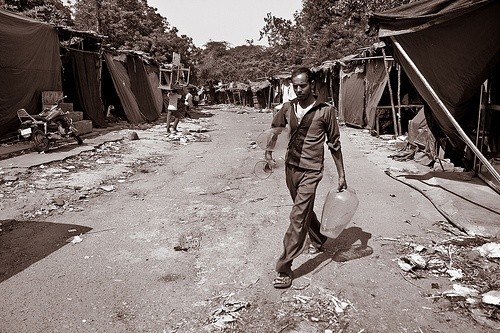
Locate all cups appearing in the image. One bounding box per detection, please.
[257,127,291,152]
[255,157,286,180]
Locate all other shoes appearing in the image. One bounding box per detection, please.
[309,242,321,253]
[272,271,292,288]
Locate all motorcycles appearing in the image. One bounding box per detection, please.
[16,96,83,152]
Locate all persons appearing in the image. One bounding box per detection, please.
[194,92,209,111]
[183,88,193,119]
[166,89,182,134]
[263,66,348,288]
